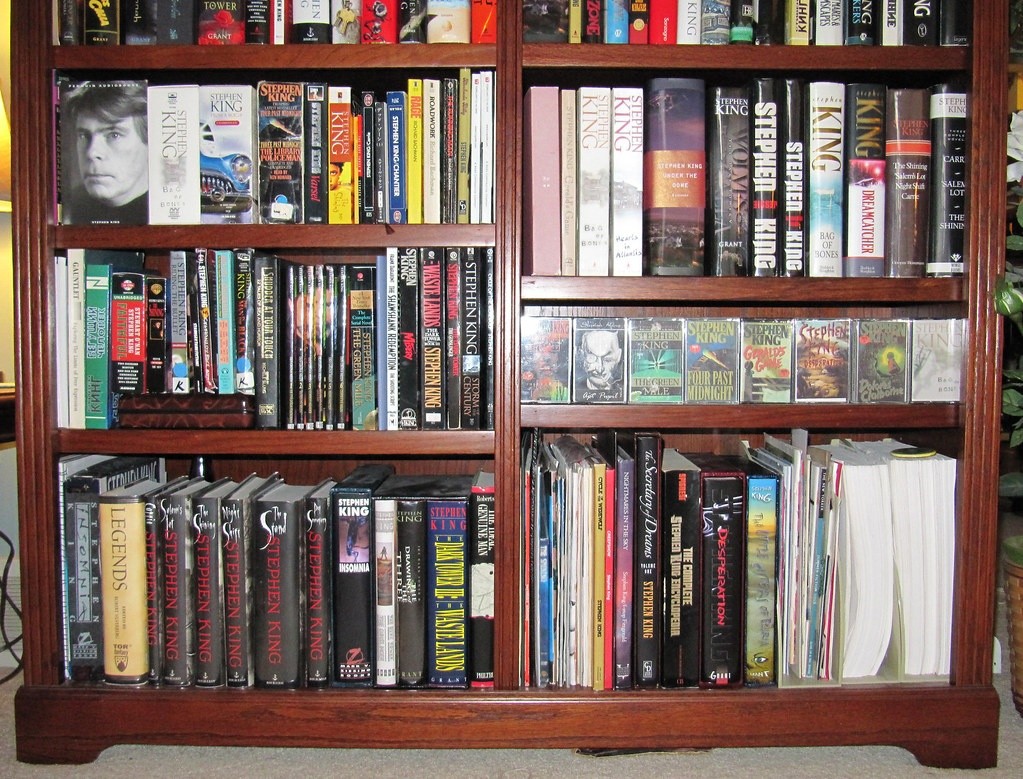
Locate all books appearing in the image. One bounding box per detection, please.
[54,0,968,691]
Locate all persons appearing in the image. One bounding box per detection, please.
[329,162,345,191]
[64,80,149,224]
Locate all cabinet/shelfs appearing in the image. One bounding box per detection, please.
[10,0,1005,767]
